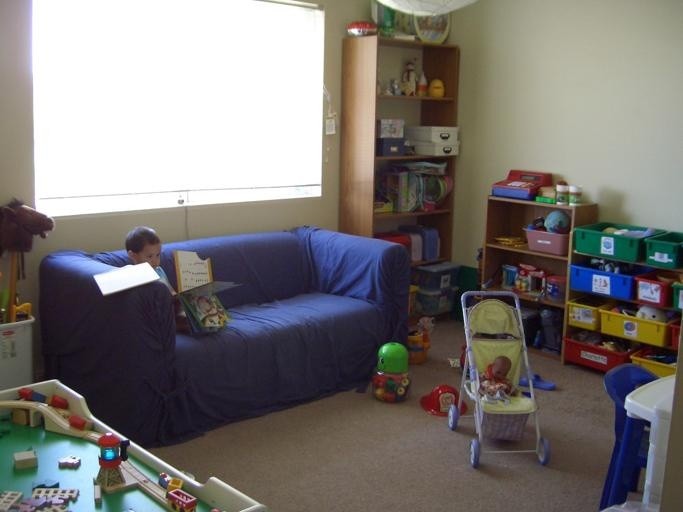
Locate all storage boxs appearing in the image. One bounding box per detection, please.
[370,0,450,44]
[561,222,683,377]
[523,225,569,256]
[409,261,461,317]
[0,314,35,415]
[377,118,461,157]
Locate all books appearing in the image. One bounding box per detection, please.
[172,249,241,337]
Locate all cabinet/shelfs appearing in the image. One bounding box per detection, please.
[480,195,599,366]
[338,35,461,326]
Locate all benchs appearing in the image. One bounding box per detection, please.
[40,225,410,450]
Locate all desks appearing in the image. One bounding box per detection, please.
[607,374,675,508]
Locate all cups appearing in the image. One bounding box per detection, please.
[555,184,569,205]
[568,185,582,207]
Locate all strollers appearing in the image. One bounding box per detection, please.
[443,288,556,469]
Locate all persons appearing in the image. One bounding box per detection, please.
[125,226,187,335]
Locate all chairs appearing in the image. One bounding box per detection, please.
[598,363,659,512]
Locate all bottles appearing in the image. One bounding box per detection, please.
[416,71,428,97]
